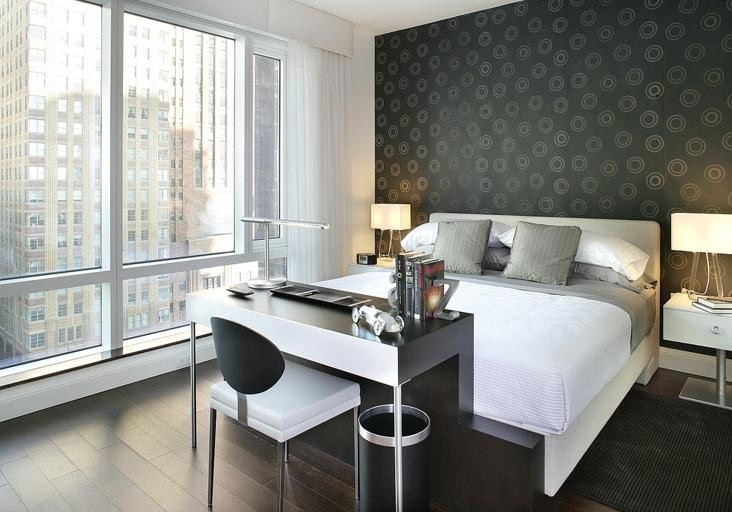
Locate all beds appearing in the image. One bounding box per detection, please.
[306,208,658,495]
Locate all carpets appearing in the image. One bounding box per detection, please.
[560,388,731,511]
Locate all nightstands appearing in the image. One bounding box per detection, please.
[346,257,396,275]
[662,293,732,409]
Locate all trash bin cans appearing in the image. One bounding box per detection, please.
[358,404,431,511]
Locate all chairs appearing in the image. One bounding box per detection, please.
[203,314,362,512]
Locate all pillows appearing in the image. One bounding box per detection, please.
[504,221,584,287]
[430,218,492,277]
[497,227,652,279]
[403,219,512,251]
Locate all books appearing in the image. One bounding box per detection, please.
[398,251,444,320]
[692,301,732,314]
[697,297,732,309]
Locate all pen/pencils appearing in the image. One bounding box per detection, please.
[707,298,732,303]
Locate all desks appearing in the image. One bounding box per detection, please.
[187,276,474,512]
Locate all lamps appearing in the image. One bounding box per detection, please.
[240,215,329,290]
[670,212,732,306]
[368,201,411,262]
